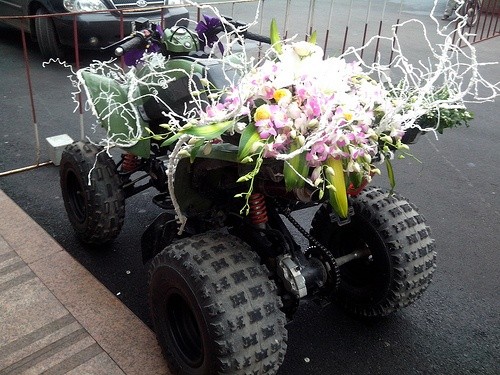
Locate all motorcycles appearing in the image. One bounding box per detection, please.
[58,13,438,375]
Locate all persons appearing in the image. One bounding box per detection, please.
[442,0,483,20]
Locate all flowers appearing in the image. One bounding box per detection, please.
[126,18,475,219]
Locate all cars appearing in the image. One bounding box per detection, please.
[0,0,187,64]
